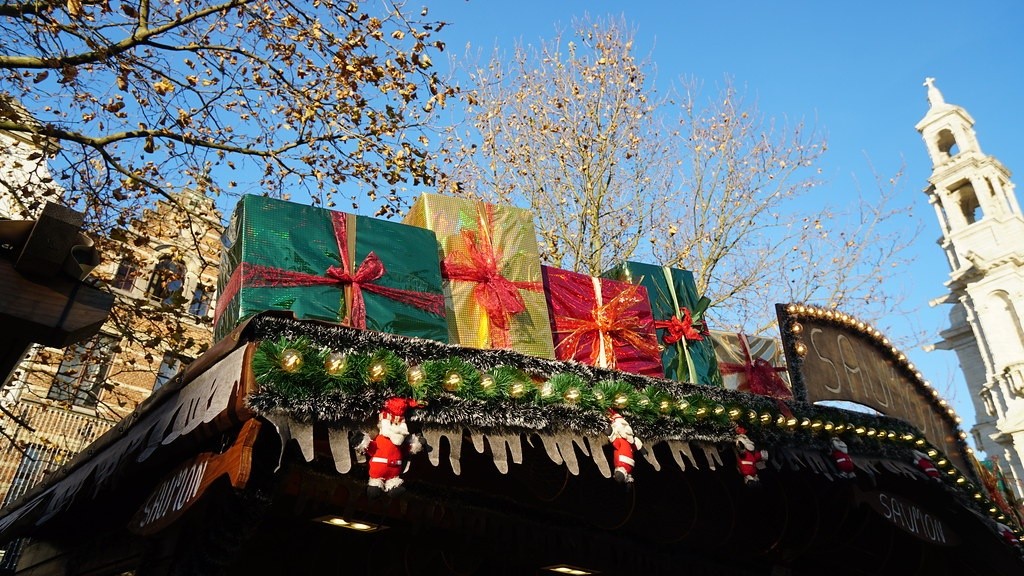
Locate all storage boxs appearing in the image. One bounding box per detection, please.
[212,191,790,399]
